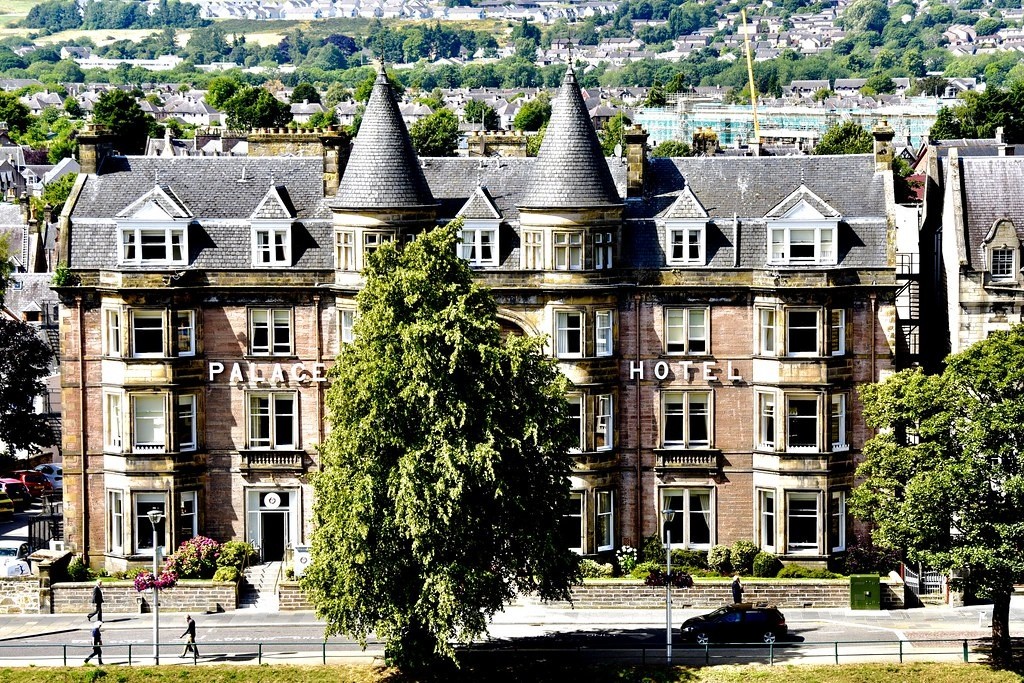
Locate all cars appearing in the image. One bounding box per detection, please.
[679,602,787,648]
[0,463,62,517]
[0,539,35,575]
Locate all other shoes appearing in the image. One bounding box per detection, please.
[193,656,199,659]
[178,656,184,658]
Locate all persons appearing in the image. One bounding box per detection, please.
[84,624,104,666]
[87,580,103,623]
[179,614,200,659]
[732,575,744,605]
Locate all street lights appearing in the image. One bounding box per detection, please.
[146,507,163,665]
[661,509,676,666]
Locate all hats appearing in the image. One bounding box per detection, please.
[733,575,739,581]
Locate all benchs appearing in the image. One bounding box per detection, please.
[48,522,63,540]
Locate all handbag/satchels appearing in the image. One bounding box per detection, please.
[91,601,97,613]
[188,641,195,651]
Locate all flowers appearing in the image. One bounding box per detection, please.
[135,569,179,591]
[646,567,693,590]
